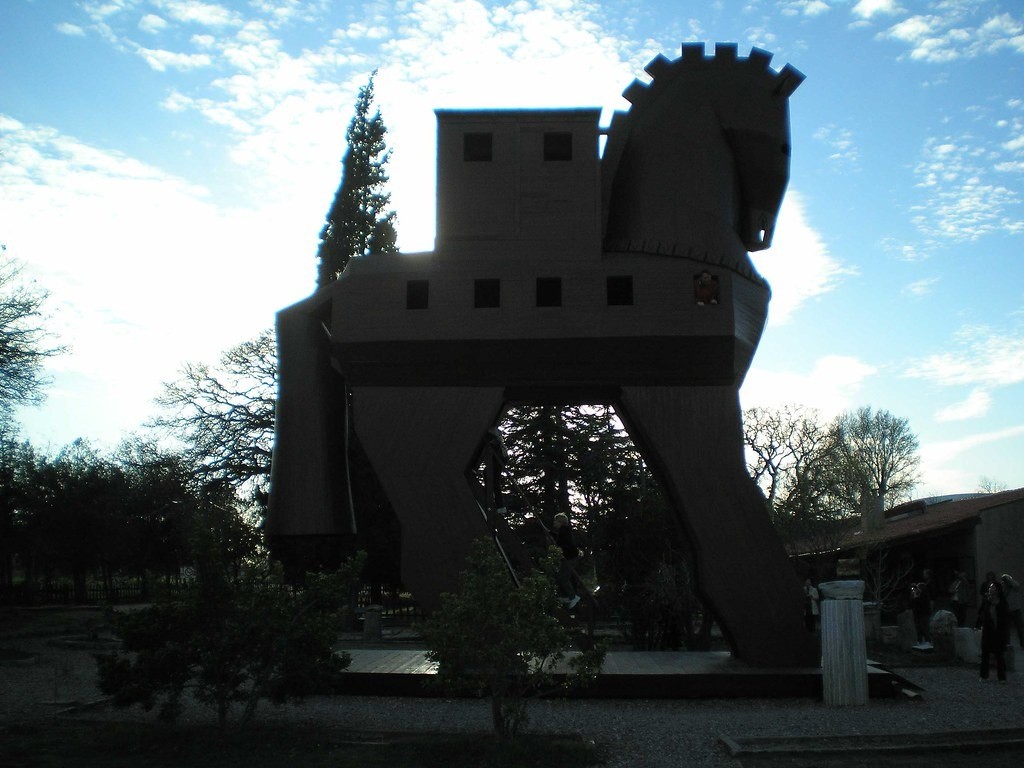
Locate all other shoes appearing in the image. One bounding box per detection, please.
[496,497,502,507]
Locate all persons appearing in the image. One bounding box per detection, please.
[802,577,819,632]
[909,569,1024,684]
[543,513,583,604]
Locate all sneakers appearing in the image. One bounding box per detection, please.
[569,595,581,610]
